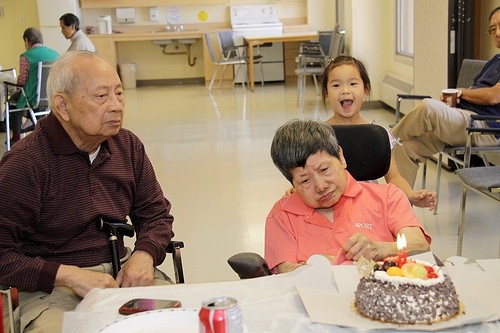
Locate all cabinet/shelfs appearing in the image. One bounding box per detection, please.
[90,38,117,73]
[202,38,234,89]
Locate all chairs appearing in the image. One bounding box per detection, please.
[228,124,392,279]
[294,24,344,108]
[389,59,500,256]
[0,61,54,152]
[0,241,185,333]
[205,30,263,91]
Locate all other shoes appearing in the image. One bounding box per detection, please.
[20,123,36,133]
[3,139,18,147]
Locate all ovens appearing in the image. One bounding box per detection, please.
[232,28,284,83]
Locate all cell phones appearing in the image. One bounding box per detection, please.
[118,298,182,315]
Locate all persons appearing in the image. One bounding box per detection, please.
[0,50,175,333]
[60,13,96,53]
[390,7,500,190]
[264,120,432,274]
[10,27,59,144]
[322,56,438,211]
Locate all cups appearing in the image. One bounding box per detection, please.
[442,88,459,108]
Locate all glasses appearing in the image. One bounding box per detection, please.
[488,22,500,35]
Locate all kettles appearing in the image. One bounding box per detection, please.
[96,15,113,34]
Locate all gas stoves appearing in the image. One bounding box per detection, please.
[230,4,282,28]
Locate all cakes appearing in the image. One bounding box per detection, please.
[355,257,459,324]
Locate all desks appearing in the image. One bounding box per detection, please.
[66,266,500,333]
[243,31,318,92]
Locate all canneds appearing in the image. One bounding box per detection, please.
[198,295,244,333]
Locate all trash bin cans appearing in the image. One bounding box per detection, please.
[119,63,136,90]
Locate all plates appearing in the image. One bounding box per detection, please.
[96,306,248,333]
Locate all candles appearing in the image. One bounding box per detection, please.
[401,233,407,264]
[397,233,402,268]
